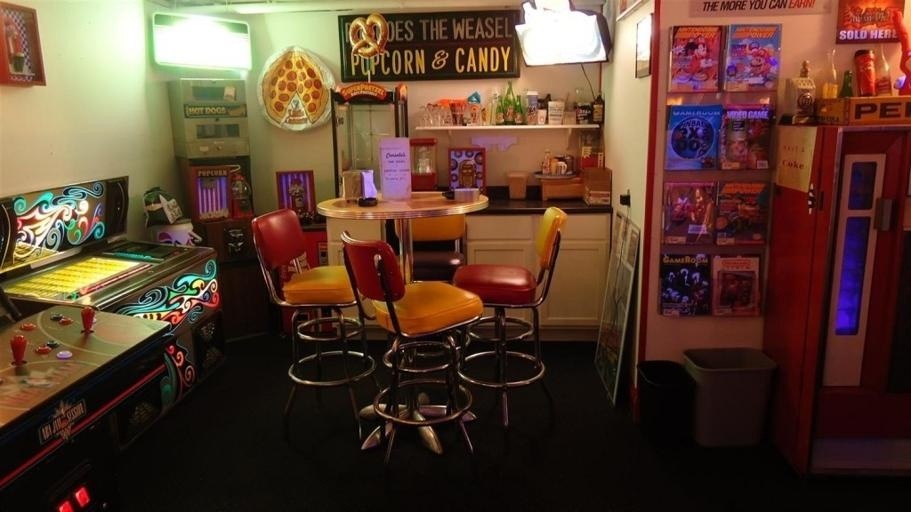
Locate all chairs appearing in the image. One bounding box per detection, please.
[386,208,474,362]
[447,202,570,455]
[249,207,388,446]
[335,228,486,463]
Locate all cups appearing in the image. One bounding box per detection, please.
[507,171,528,200]
[416,100,498,128]
[854,49,877,98]
[536,109,548,124]
[524,88,539,126]
[548,101,564,126]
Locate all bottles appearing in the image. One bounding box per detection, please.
[872,42,894,98]
[819,47,840,99]
[538,148,575,177]
[494,80,529,126]
[573,87,592,124]
[591,92,605,124]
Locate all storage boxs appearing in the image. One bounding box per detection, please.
[580,165,613,208]
[813,94,910,126]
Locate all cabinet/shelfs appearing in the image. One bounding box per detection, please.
[324,214,391,330]
[771,119,910,481]
[468,209,615,345]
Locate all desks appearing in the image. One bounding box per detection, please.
[315,188,490,465]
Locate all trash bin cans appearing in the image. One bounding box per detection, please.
[637,360,690,429]
[682,348,777,448]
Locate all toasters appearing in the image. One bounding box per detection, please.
[835,68,854,99]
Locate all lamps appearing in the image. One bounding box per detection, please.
[150,9,256,76]
[511,0,614,70]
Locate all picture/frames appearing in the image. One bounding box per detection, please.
[0,2,47,88]
[634,12,654,80]
[447,145,489,197]
